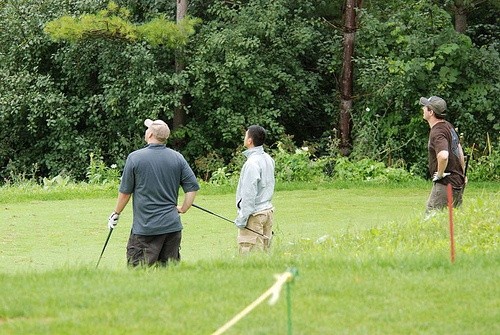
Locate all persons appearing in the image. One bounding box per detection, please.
[234,125,275,254]
[420,96,469,220]
[108,119,200,269]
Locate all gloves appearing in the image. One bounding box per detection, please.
[233,216,248,229]
[108,211,119,230]
[464,176,468,185]
[432,171,451,182]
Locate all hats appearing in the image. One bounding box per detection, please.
[144,119,170,141]
[420,96,448,115]
